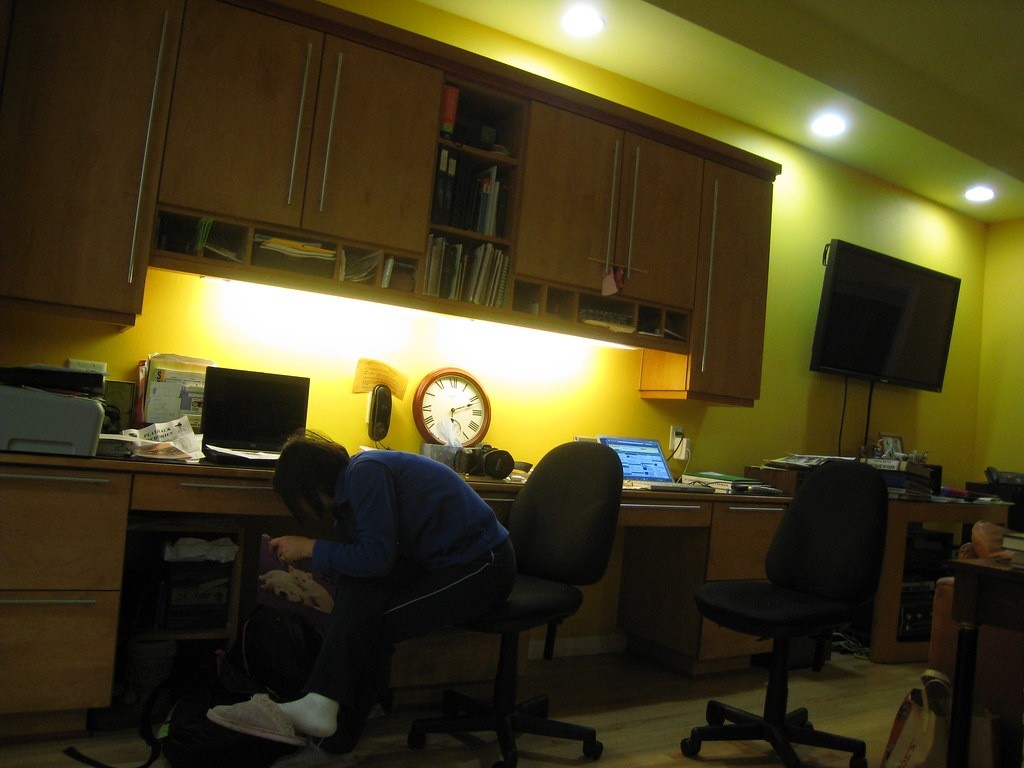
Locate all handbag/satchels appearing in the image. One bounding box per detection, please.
[880,669,997,768]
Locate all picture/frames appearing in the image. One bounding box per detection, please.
[878,432,905,458]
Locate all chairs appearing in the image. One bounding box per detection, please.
[404,441,623,768]
[680,459,891,768]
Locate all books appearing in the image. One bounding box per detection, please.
[1000,529,1024,567]
[253,232,336,263]
[418,231,509,307]
[433,147,500,236]
[436,82,461,141]
[681,470,764,495]
[137,353,208,428]
[254,533,337,635]
[760,452,1003,504]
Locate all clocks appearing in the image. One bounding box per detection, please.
[412,369,492,445]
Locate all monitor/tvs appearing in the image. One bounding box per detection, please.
[809,238,962,394]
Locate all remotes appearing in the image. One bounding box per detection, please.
[747,485,782,496]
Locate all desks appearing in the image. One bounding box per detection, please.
[0,446,788,733]
[945,556,1024,768]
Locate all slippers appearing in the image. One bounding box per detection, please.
[207,693,310,746]
[270,740,361,768]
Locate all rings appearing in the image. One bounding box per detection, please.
[280,555,286,561]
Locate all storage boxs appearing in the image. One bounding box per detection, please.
[143,552,234,630]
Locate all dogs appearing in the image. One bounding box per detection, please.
[259,569,301,603]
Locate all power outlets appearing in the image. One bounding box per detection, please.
[670,426,685,448]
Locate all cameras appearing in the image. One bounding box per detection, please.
[454,444,514,480]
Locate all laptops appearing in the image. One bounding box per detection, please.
[202,366,310,466]
[596,436,716,494]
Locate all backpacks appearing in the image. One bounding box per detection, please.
[142,608,325,768]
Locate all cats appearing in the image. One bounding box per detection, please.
[287,565,334,614]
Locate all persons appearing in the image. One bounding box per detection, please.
[207,430,518,768]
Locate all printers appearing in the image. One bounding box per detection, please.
[0,363,133,460]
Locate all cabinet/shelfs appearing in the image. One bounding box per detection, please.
[744,465,944,497]
[0,0,169,328]
[152,0,443,301]
[961,481,1024,544]
[414,34,534,322]
[500,63,700,345]
[686,135,780,400]
[867,497,1008,659]
